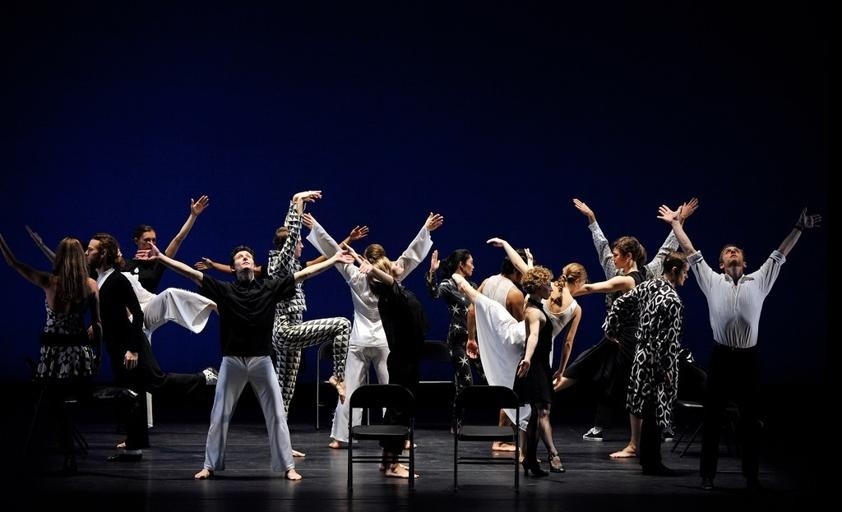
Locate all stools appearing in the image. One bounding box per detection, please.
[666,395,744,458]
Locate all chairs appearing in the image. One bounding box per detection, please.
[419,341,458,384]
[444,382,523,491]
[342,381,418,497]
[314,337,374,430]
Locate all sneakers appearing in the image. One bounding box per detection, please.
[583,428,603,441]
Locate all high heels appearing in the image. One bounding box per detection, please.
[522,454,565,476]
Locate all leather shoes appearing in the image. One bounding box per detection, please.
[108,453,141,461]
[203,366,218,385]
